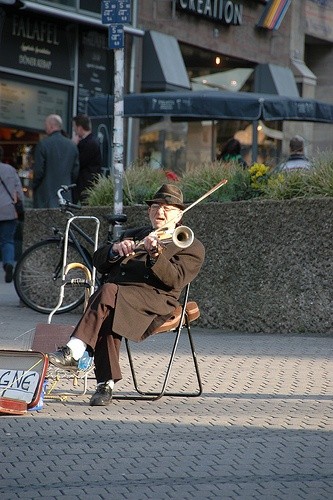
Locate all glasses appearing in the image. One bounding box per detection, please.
[151,203,176,211]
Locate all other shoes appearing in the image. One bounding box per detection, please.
[4,264,13,283]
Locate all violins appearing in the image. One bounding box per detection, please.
[149,227,170,256]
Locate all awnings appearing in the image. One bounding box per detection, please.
[88,90,333,165]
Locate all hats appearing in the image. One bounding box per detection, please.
[145,184,186,211]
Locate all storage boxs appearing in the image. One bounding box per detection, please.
[0,349,50,414]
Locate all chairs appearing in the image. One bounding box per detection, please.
[110,282,203,401]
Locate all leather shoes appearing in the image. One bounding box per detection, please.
[90,384,113,406]
[44,344,80,373]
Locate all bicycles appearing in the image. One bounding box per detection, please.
[13,183,129,314]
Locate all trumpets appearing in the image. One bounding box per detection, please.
[172,226,195,250]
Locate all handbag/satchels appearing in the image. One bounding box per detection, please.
[14,200,22,217]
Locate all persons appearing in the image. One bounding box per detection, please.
[279,135,312,169]
[0,161,24,283]
[25,115,80,208]
[71,116,101,205]
[45,183,205,406]
[217,139,249,170]
